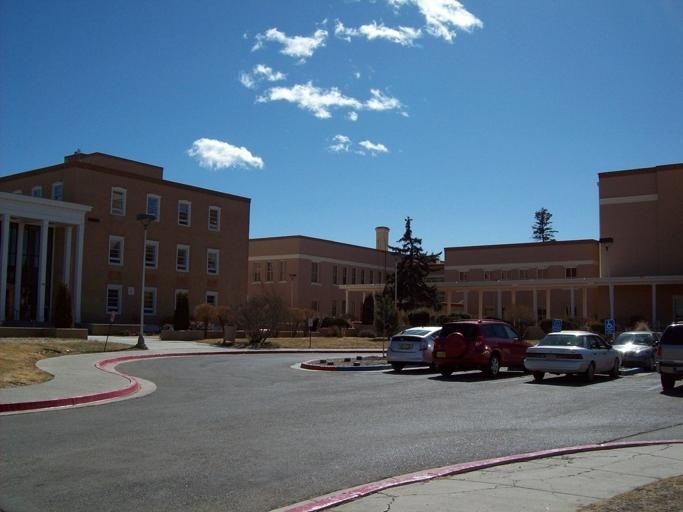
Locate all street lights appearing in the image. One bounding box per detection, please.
[134,213,156,347]
[288,273,295,334]
[390,252,402,329]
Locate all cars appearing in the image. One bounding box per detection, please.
[612,331,663,371]
[385,326,442,370]
[522,329,621,382]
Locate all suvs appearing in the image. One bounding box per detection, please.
[655,322,682,390]
[431,319,533,379]
